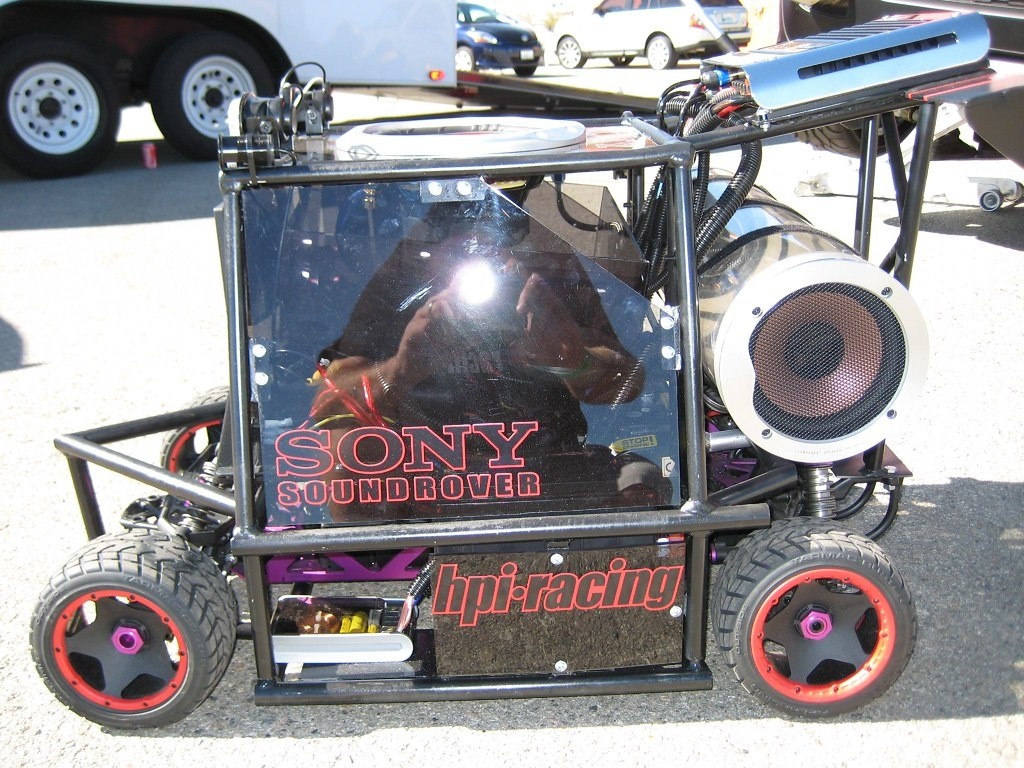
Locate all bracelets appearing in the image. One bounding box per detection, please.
[558,350,592,382]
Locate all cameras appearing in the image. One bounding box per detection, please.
[454,276,526,330]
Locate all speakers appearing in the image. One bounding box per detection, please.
[687,167,930,464]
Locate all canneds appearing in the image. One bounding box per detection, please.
[141,143,157,169]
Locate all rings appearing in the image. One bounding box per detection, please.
[427,299,437,320]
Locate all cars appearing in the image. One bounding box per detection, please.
[455,1,543,77]
[552,0,752,72]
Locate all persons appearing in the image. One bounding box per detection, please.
[308,173,674,520]
